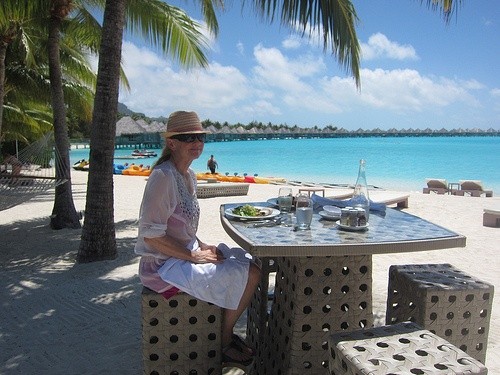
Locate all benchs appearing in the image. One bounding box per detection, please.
[323,190,354,200]
[376,193,410,207]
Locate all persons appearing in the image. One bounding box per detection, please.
[3,153,23,177]
[124,163,150,170]
[82,159,88,164]
[208,155,216,173]
[135,111,261,367]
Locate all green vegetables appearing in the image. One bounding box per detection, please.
[232,204,261,216]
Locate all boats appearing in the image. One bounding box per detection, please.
[73,159,285,183]
[131,149,158,156]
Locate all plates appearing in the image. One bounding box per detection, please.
[318,211,341,221]
[323,205,342,217]
[224,205,281,219]
[336,220,369,231]
[267,198,314,208]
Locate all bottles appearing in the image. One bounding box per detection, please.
[352,159,369,222]
[340,206,366,227]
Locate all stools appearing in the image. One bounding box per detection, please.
[390,262,495,362]
[248,256,279,351]
[140,284,223,375]
[326,324,487,375]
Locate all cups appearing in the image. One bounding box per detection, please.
[278,186,292,213]
[295,198,313,229]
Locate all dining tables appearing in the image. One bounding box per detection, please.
[218,203,465,375]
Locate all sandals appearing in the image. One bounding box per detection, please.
[232,335,254,355]
[223,343,253,366]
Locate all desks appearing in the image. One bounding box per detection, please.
[299,187,325,196]
[448,182,460,194]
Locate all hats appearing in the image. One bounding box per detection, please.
[159,110,212,138]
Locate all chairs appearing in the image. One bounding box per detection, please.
[482,207,500,227]
[195,182,253,199]
[423,179,456,194]
[456,180,493,197]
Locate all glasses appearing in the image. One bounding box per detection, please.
[169,134,206,142]
